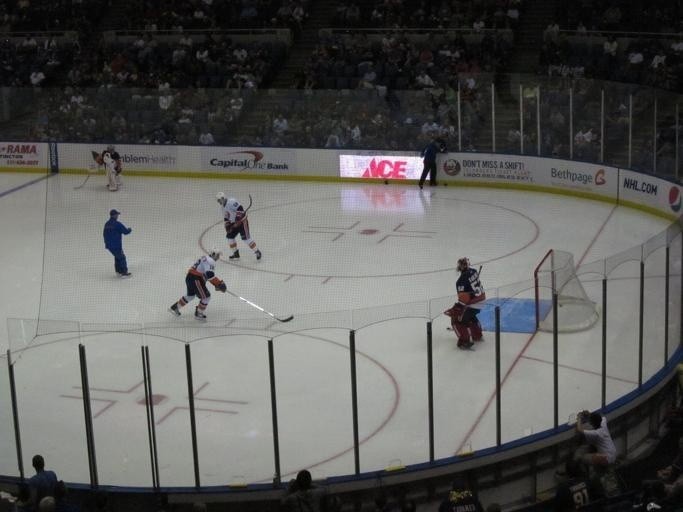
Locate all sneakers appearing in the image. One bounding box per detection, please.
[555,469,566,479]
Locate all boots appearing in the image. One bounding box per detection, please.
[229,249,240,258]
[418,182,437,189]
[170,302,181,315]
[194,306,207,318]
[255,248,261,260]
[115,268,132,278]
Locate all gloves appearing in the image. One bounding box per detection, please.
[226,216,241,232]
[215,280,227,293]
[444,302,464,317]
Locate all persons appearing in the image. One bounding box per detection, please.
[192,501,207,512]
[1,3,523,151]
[419,139,443,189]
[96,145,123,190]
[631,363,683,511]
[281,469,416,511]
[507,4,682,176]
[444,258,486,348]
[170,251,226,319]
[439,477,501,512]
[557,411,618,512]
[215,192,261,260]
[26,454,58,512]
[103,210,132,278]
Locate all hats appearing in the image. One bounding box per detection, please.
[110,209,120,215]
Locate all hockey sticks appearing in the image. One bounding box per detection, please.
[232,196,252,225]
[447,266,482,330]
[74,175,90,189]
[226,289,294,322]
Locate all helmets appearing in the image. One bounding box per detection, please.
[210,248,223,260]
[216,192,228,205]
[456,256,471,272]
